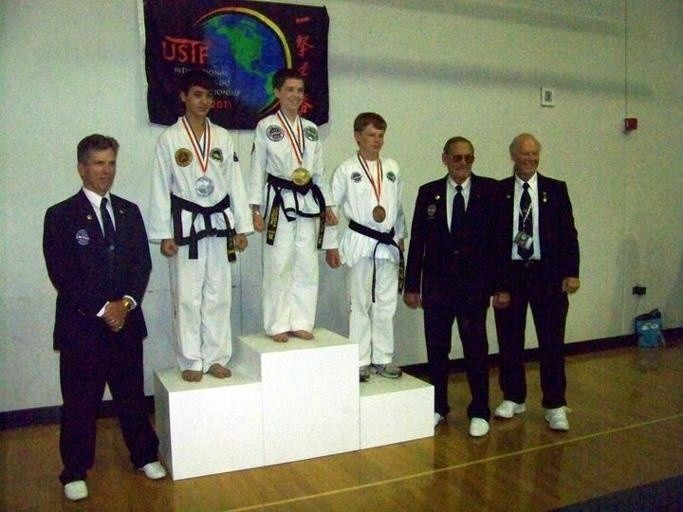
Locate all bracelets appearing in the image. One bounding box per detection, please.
[122,298,132,313]
[251,210,262,217]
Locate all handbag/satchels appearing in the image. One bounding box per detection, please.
[635,309,666,348]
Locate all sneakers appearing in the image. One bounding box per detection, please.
[370,364,402,378]
[358,363,371,382]
[545,406,570,431]
[469,417,489,436]
[63,480,87,500]
[138,461,166,479]
[434,412,445,427]
[494,400,526,418]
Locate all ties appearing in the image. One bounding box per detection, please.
[518,183,533,261]
[100,198,115,254]
[451,186,465,239]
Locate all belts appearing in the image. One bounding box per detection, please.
[513,260,541,273]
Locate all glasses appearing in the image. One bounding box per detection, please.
[448,152,474,163]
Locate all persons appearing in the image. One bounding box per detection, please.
[490,133,580,432]
[248,67,339,347]
[321,110,410,383]
[401,136,510,435]
[146,68,256,384]
[42,132,167,503]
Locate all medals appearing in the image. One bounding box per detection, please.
[373,206,386,223]
[195,176,214,196]
[292,167,311,186]
